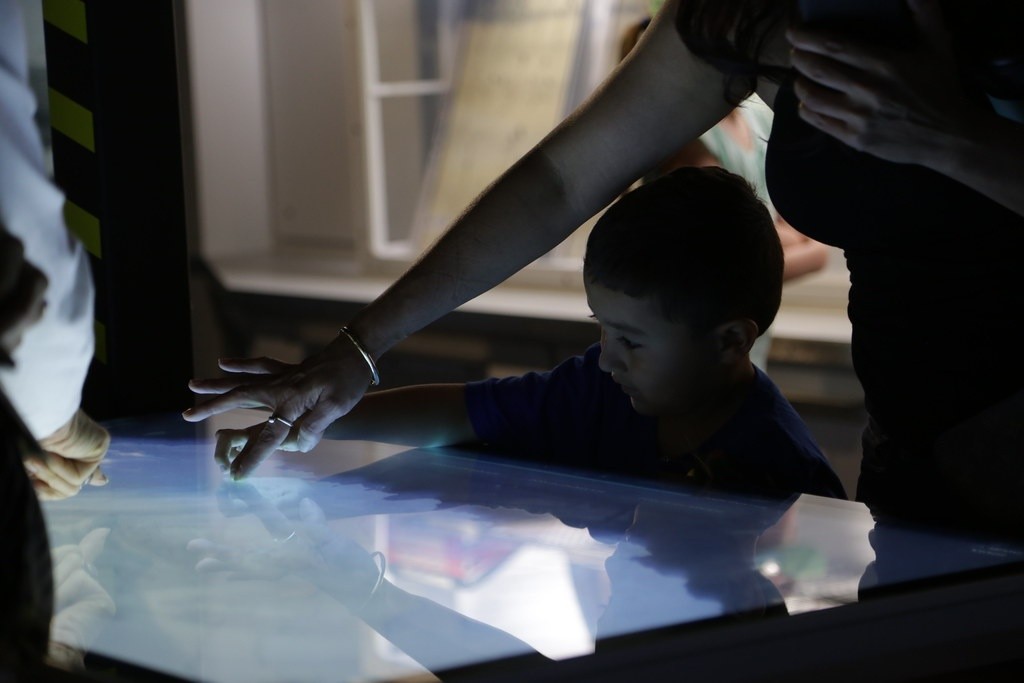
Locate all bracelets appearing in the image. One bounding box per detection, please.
[335,313,388,394]
[335,542,385,621]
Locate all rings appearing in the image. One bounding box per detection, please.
[266,410,295,429]
[275,528,303,555]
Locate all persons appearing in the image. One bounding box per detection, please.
[162,513,826,683]
[30,520,128,670]
[2,0,138,498]
[210,474,842,683]
[0,232,86,683]
[185,424,807,673]
[600,6,826,397]
[186,163,854,532]
[164,0,1024,683]
